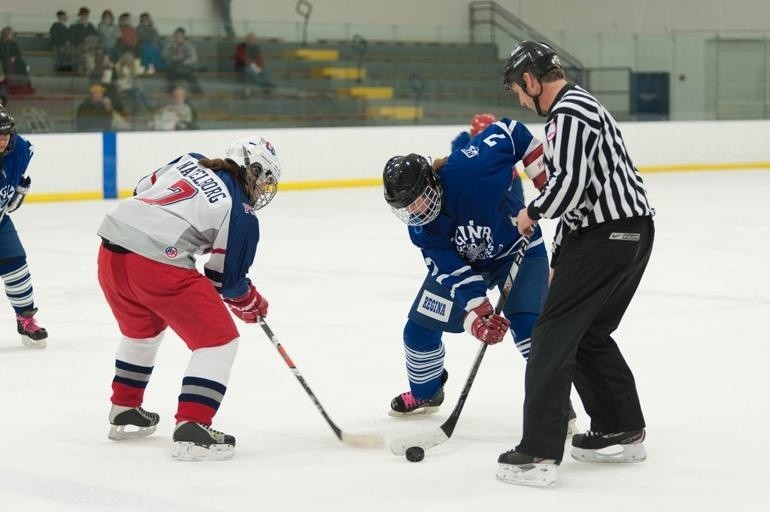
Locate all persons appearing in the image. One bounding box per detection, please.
[97,137,280,449]
[497,40,654,466]
[0,103,48,340]
[451,115,524,205]
[382,117,577,425]
[1,8,275,130]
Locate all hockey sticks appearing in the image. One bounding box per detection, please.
[389,219,539,456]
[255,315,385,449]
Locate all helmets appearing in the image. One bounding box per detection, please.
[502,39,562,93]
[0,105,17,158]
[470,113,495,139]
[383,152,442,228]
[226,137,281,213]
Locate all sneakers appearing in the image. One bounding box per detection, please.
[108,403,161,428]
[172,419,236,446]
[572,423,646,448]
[391,388,445,412]
[16,307,48,342]
[497,444,562,466]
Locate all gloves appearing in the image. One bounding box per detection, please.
[8,178,30,214]
[224,281,269,325]
[464,295,512,345]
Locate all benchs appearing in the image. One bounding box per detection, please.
[0,30,549,134]
